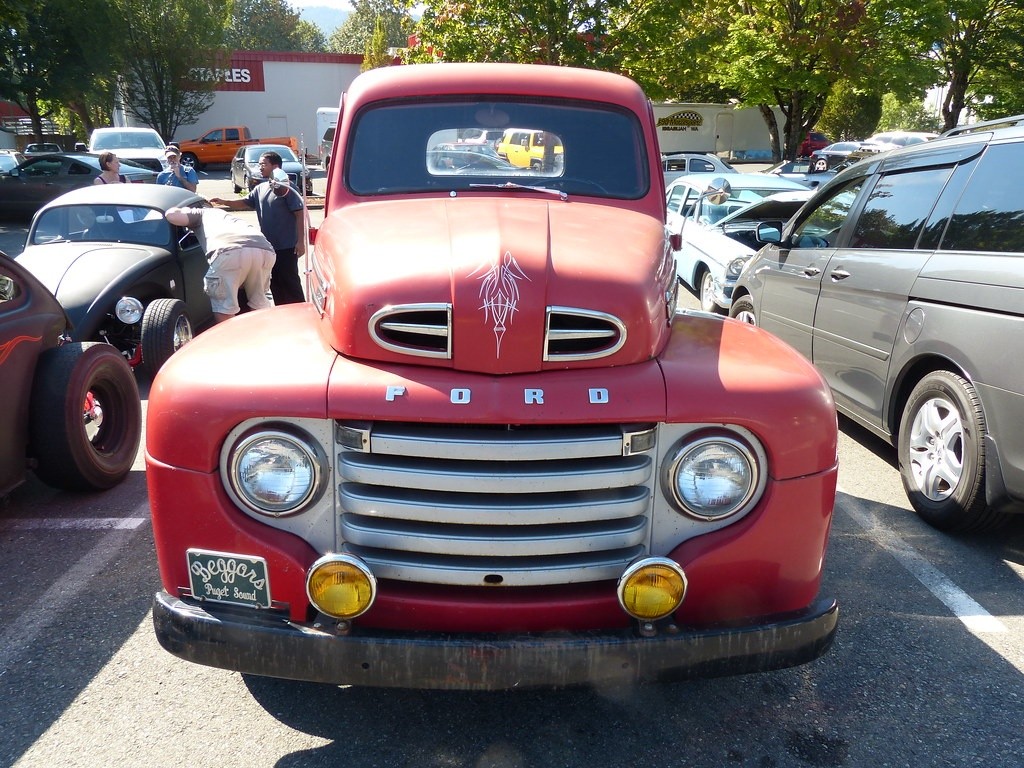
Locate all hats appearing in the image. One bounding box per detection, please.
[165,145,179,158]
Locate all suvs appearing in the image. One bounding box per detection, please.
[725,112,1024,537]
[319,125,337,174]
[74,127,181,174]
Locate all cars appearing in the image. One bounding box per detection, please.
[0,148,34,176]
[23,142,64,169]
[142,60,844,694]
[427,127,564,178]
[14,181,214,384]
[0,250,101,502]
[230,143,314,196]
[0,151,163,220]
[662,130,940,317]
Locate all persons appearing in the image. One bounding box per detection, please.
[94,152,133,211]
[209,151,306,307]
[156,144,199,192]
[165,207,276,324]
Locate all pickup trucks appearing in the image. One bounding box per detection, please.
[178,125,300,173]
